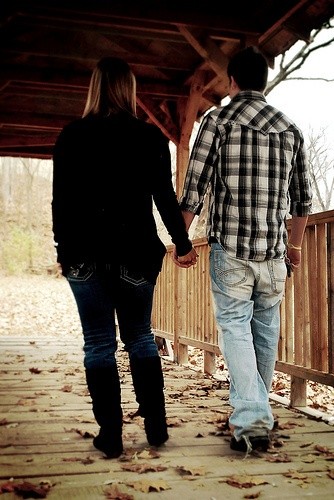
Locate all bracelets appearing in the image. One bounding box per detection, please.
[287,242,301,250]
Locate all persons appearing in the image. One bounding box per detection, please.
[52,58,197,458]
[173,44,311,454]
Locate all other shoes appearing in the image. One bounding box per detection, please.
[145,421,169,446]
[92,436,124,460]
[229,435,271,452]
